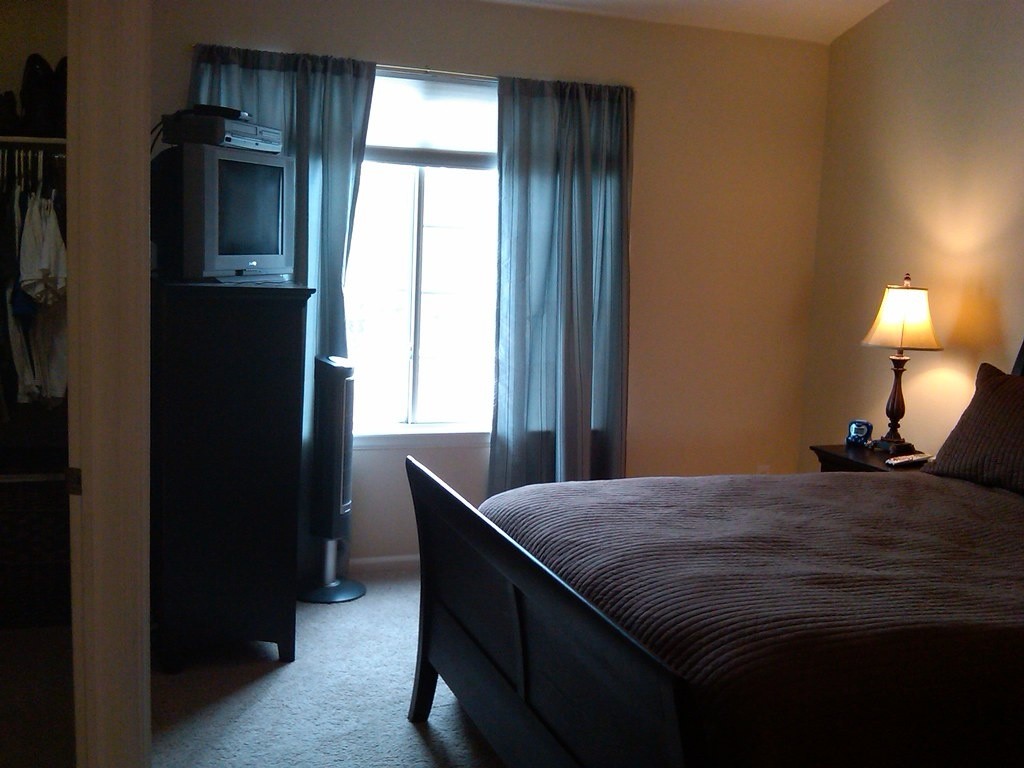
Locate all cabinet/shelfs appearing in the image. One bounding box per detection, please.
[0,137,81,628]
[150,281,316,662]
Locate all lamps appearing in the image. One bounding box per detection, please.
[861,273,944,451]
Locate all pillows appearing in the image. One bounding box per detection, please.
[920,362,1024,493]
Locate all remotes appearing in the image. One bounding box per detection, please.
[886,454,932,467]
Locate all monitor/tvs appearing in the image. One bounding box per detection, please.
[151,142,297,282]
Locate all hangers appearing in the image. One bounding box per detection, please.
[0,149,44,198]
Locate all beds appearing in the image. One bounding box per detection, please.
[406,341,1024,768]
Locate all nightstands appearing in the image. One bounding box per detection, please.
[810,445,928,473]
